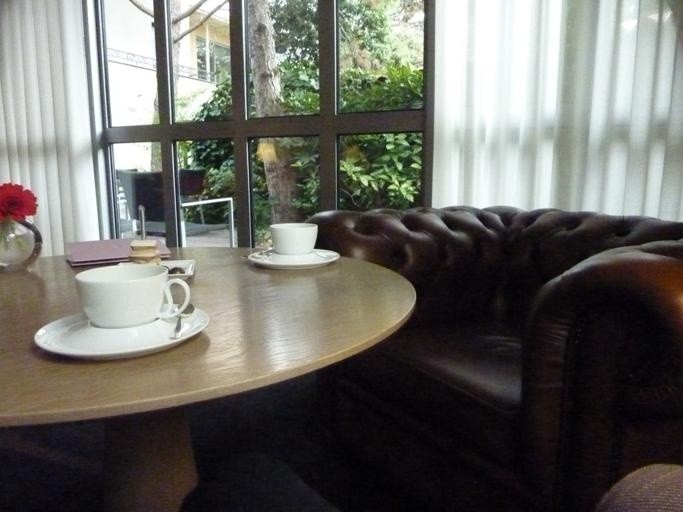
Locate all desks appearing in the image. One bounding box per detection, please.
[0,247,417,512]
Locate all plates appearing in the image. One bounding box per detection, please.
[249,249,341,270]
[120,260,196,281]
[33,307,208,360]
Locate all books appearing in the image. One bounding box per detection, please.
[66,237,171,267]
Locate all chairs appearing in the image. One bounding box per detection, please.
[115,166,207,240]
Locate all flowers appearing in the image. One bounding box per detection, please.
[0,183,38,251]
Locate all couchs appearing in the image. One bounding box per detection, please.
[296,203,682,511]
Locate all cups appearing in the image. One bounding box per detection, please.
[270,222,319,254]
[76,264,190,329]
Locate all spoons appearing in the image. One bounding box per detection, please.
[170,302,195,339]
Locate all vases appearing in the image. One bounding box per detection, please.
[0,219,42,275]
